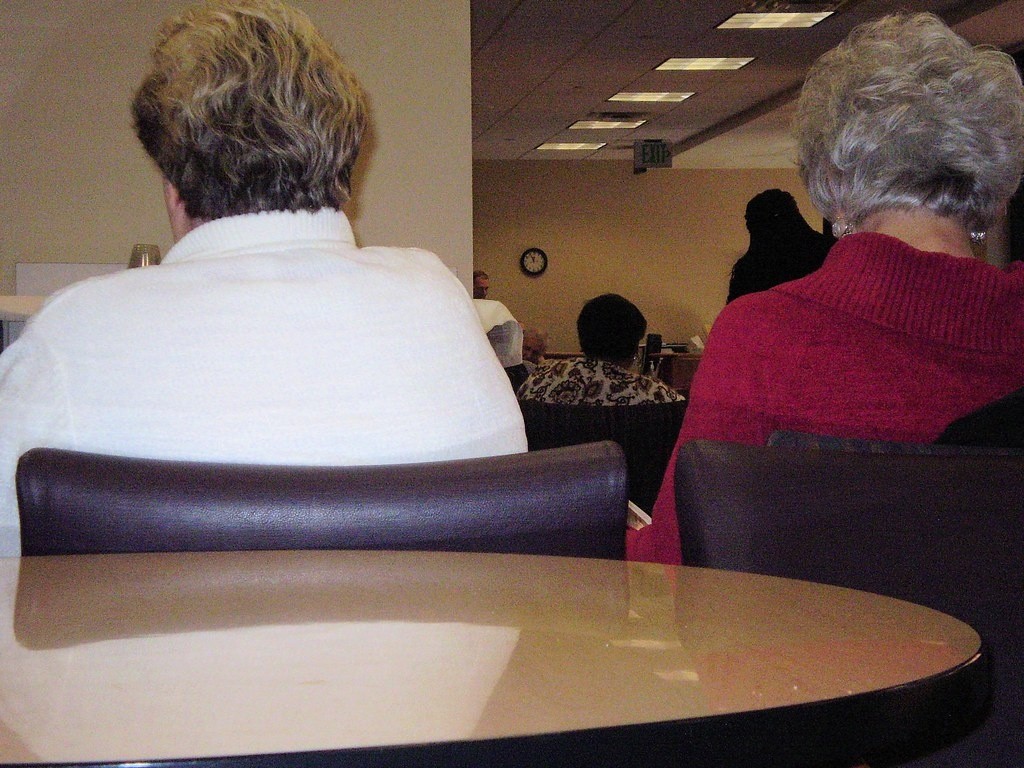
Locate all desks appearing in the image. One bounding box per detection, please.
[0,549,993,768]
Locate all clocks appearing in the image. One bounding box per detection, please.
[520,248,548,276]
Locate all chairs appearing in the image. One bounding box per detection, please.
[13,353,1024,768]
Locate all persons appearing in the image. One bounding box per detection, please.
[0,0,528,556]
[624,13,1024,567]
[725,188,838,305]
[518,320,547,365]
[473,269,489,300]
[516,294,688,408]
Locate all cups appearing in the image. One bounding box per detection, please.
[128,244,162,269]
